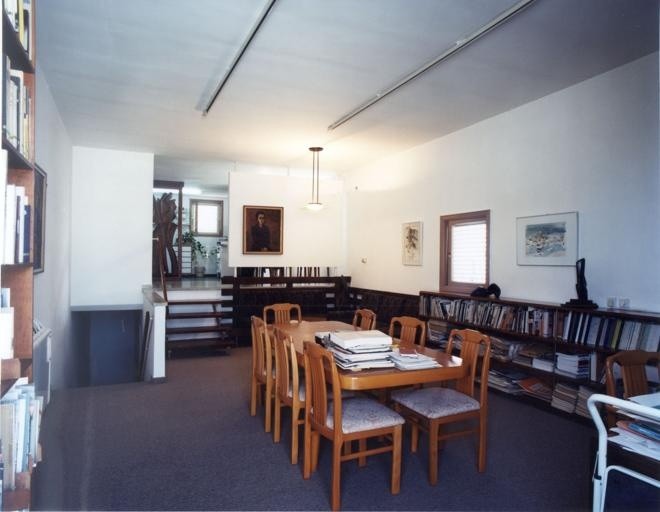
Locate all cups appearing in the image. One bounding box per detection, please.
[619,297,630,309]
[607,296,616,308]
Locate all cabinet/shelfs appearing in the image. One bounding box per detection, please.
[416,291,660,426]
[2,0,33,510]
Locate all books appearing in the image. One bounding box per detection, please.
[419,294,659,422]
[1,0,51,510]
[606,393,659,459]
[324,330,443,372]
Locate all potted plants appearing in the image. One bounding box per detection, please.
[176,232,207,279]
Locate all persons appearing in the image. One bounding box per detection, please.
[250,211,270,252]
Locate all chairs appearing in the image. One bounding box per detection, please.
[605,352,659,433]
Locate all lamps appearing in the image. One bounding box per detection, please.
[307,146,324,210]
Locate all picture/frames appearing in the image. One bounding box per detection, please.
[516,212,579,266]
[242,205,283,255]
[34,162,47,273]
[401,221,424,266]
